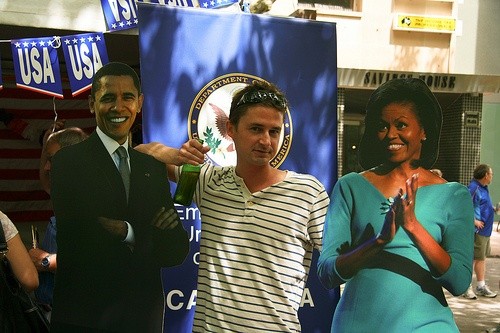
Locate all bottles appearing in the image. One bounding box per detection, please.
[172,138,204,206]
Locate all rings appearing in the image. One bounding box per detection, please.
[407,199,412,205]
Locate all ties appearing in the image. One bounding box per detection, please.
[116,146,130,207]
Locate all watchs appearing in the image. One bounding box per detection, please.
[42,255,50,272]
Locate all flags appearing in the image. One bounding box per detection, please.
[137,3,336,333]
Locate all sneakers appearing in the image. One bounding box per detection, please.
[475,285,497,299]
[462,287,477,300]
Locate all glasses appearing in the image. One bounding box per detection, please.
[233,89,287,112]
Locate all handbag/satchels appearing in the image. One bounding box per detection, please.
[0,255,51,333]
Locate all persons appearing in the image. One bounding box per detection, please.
[461,165,500,299]
[0,121,89,333]
[51,63,190,333]
[317,79,474,333]
[132,81,330,333]
